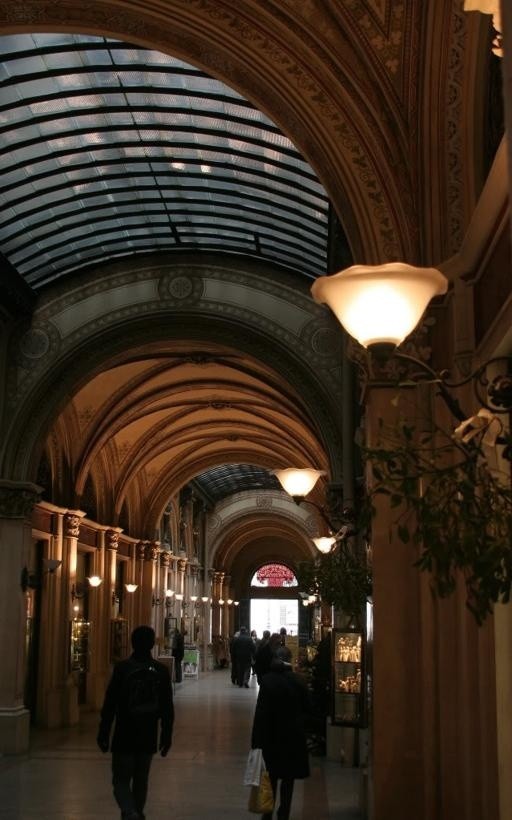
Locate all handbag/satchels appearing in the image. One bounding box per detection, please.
[249,770,273,813]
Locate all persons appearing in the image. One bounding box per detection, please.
[309,639,331,756]
[229,626,286,688]
[171,629,184,682]
[97,626,175,820]
[251,647,312,820]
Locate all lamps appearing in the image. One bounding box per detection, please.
[21,557,63,594]
[310,536,336,553]
[310,259,512,499]
[112,582,240,612]
[269,467,375,548]
[71,576,105,601]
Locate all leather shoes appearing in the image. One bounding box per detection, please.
[232,676,249,688]
